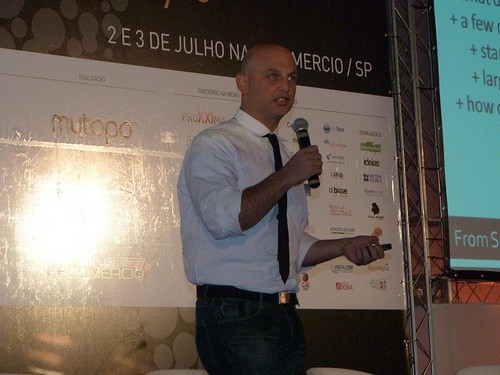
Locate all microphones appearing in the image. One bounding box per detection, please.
[291,117,321,189]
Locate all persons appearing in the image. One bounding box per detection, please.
[173,44,386,375]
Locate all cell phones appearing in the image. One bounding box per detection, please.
[365,244,392,251]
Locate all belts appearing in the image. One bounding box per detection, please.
[194,282,302,310]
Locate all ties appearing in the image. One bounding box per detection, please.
[264,131,293,287]
